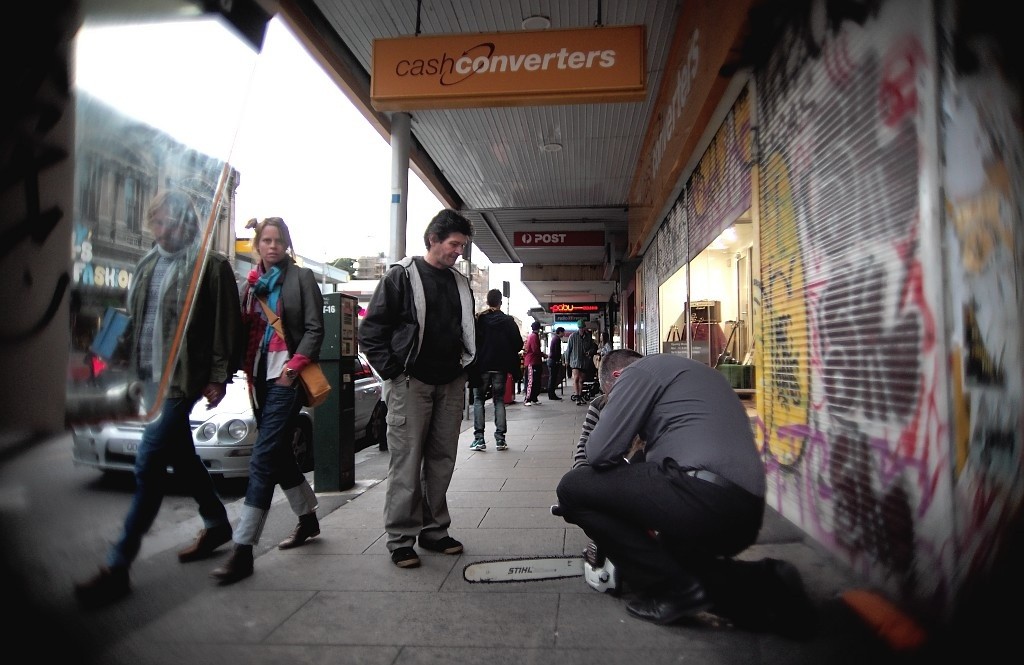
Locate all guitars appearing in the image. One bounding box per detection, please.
[715,319,744,370]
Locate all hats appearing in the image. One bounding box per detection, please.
[532,322,540,330]
[578,319,586,328]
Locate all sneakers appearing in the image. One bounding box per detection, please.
[279,512,320,550]
[496,439,508,451]
[208,545,253,586]
[470,439,486,450]
[178,522,232,562]
[418,534,463,554]
[76,567,129,606]
[390,547,420,568]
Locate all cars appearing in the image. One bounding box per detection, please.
[67,343,384,488]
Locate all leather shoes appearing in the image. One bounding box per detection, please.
[624,584,715,625]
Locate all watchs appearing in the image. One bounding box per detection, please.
[286,367,298,379]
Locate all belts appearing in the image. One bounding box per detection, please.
[685,470,730,490]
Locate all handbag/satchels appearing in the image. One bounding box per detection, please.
[299,363,332,407]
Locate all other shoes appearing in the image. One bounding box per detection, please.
[524,400,531,406]
[576,396,586,404]
[549,396,562,400]
[533,401,541,405]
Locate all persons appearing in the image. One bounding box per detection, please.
[358,209,474,567]
[200,218,321,581]
[72,188,246,604]
[469,289,524,451]
[550,391,646,591]
[556,348,767,627]
[517,319,613,406]
